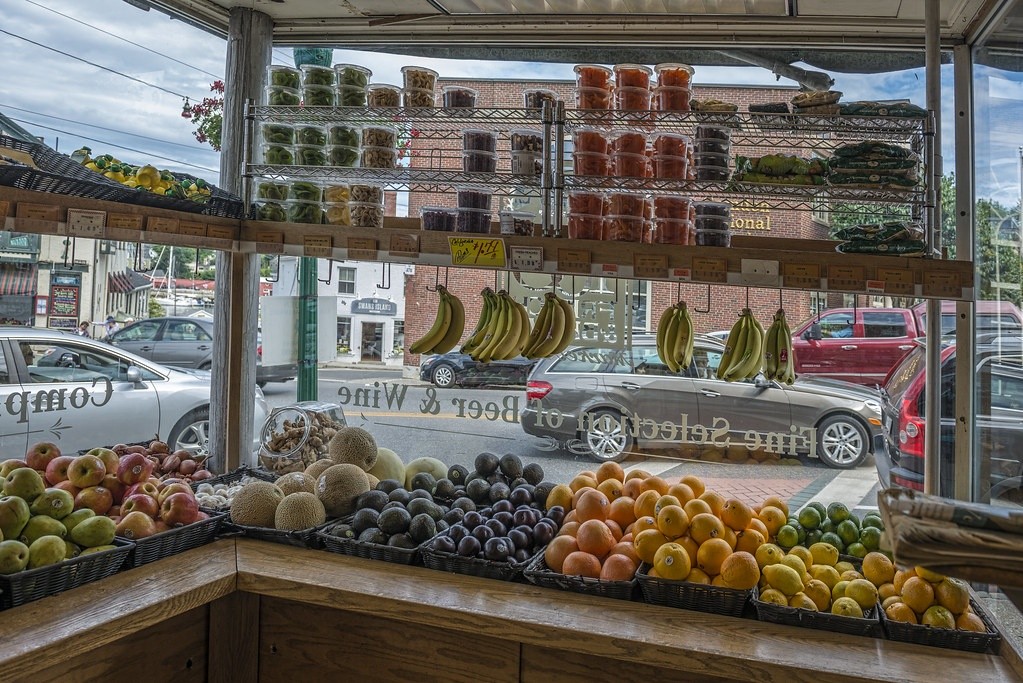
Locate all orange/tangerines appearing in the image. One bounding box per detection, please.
[546,461,790,592]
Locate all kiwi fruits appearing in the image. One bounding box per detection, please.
[257,67,365,226]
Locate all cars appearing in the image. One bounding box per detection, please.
[0,323,271,465]
[419,348,539,389]
[644,329,773,368]
[36,315,297,389]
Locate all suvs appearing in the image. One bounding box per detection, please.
[872,325,1022,513]
[520,332,883,469]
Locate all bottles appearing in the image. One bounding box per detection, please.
[259,401,349,478]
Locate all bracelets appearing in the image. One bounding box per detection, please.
[824,329,827,333]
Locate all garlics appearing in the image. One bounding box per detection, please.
[195,475,261,509]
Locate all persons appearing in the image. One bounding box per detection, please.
[124,318,133,328]
[16,342,66,383]
[88,315,120,337]
[821,316,853,338]
[72,321,90,338]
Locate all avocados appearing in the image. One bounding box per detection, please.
[330,452,559,548]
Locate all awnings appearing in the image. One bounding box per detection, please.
[126,266,154,294]
[0,264,39,297]
[108,271,135,293]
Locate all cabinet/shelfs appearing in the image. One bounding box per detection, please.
[0,182,237,683]
[237,216,1023,683]
[243,96,552,238]
[555,100,937,256]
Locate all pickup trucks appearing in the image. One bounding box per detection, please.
[788,300,1022,388]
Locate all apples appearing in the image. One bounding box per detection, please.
[25,441,62,470]
[0,448,212,541]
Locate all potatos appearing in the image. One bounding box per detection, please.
[110,441,212,481]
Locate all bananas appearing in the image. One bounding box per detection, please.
[655,302,796,384]
[409,285,575,364]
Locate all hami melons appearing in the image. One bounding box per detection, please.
[230,425,448,531]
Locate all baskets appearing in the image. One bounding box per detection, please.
[186,466,1001,653]
[0,112,261,221]
[0,504,225,614]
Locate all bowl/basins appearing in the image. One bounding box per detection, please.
[571,126,733,182]
[521,88,560,120]
[259,122,400,170]
[508,128,544,175]
[264,65,403,107]
[562,190,731,249]
[419,186,493,233]
[496,210,536,236]
[401,67,439,117]
[460,127,498,174]
[574,65,694,131]
[441,85,478,118]
[250,176,385,226]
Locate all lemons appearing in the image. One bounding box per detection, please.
[754,542,985,632]
[776,502,891,563]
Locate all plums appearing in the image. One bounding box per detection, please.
[432,500,565,564]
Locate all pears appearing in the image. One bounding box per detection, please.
[0,458,117,576]
